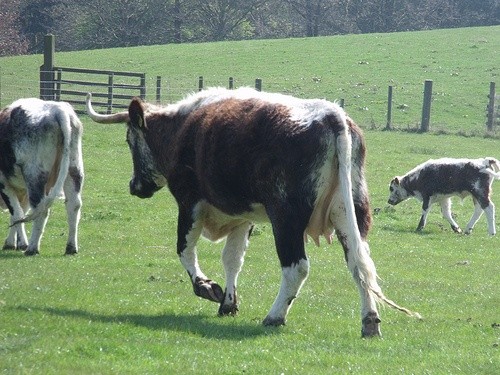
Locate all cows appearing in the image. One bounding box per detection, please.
[387,157,500,236]
[86,85,422,339]
[0,97,84,257]
